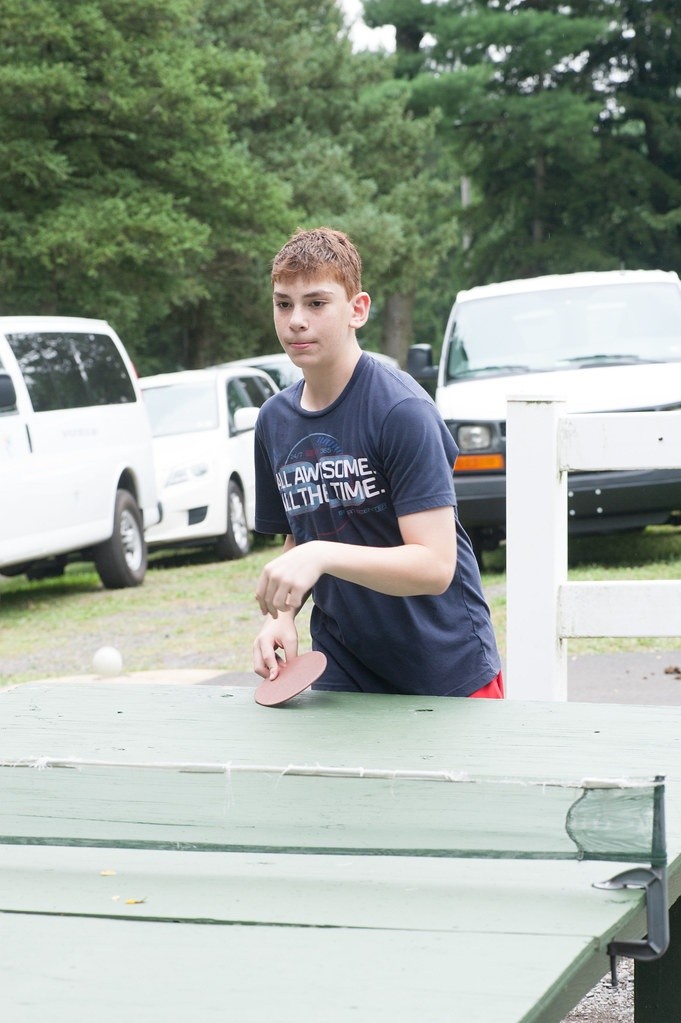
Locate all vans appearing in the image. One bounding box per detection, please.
[405,268,681,573]
[0,312,167,593]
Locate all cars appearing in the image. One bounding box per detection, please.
[133,366,284,564]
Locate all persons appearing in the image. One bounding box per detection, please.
[253,226,504,702]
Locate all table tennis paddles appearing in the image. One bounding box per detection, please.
[253,650,329,708]
